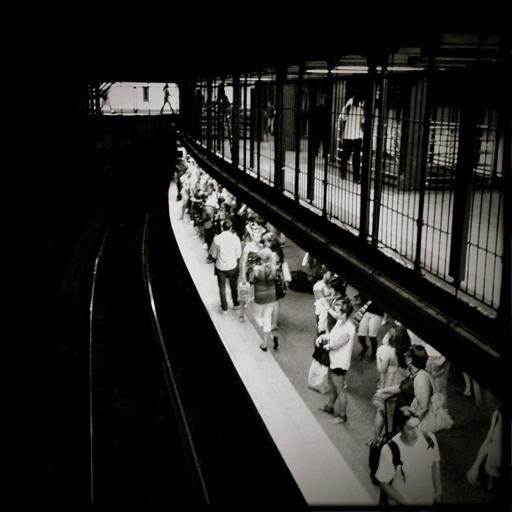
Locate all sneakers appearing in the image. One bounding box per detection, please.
[220,306,241,314]
[320,404,333,414]
[358,346,377,362]
[333,416,346,424]
[260,336,277,351]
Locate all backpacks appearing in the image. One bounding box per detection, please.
[369,431,434,487]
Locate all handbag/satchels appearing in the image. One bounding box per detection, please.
[277,280,287,299]
[289,270,310,293]
[312,344,331,367]
[238,282,249,302]
[308,358,330,394]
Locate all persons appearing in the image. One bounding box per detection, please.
[264,101,276,133]
[337,100,365,184]
[174,147,292,351]
[301,252,502,507]
[159,84,176,115]
